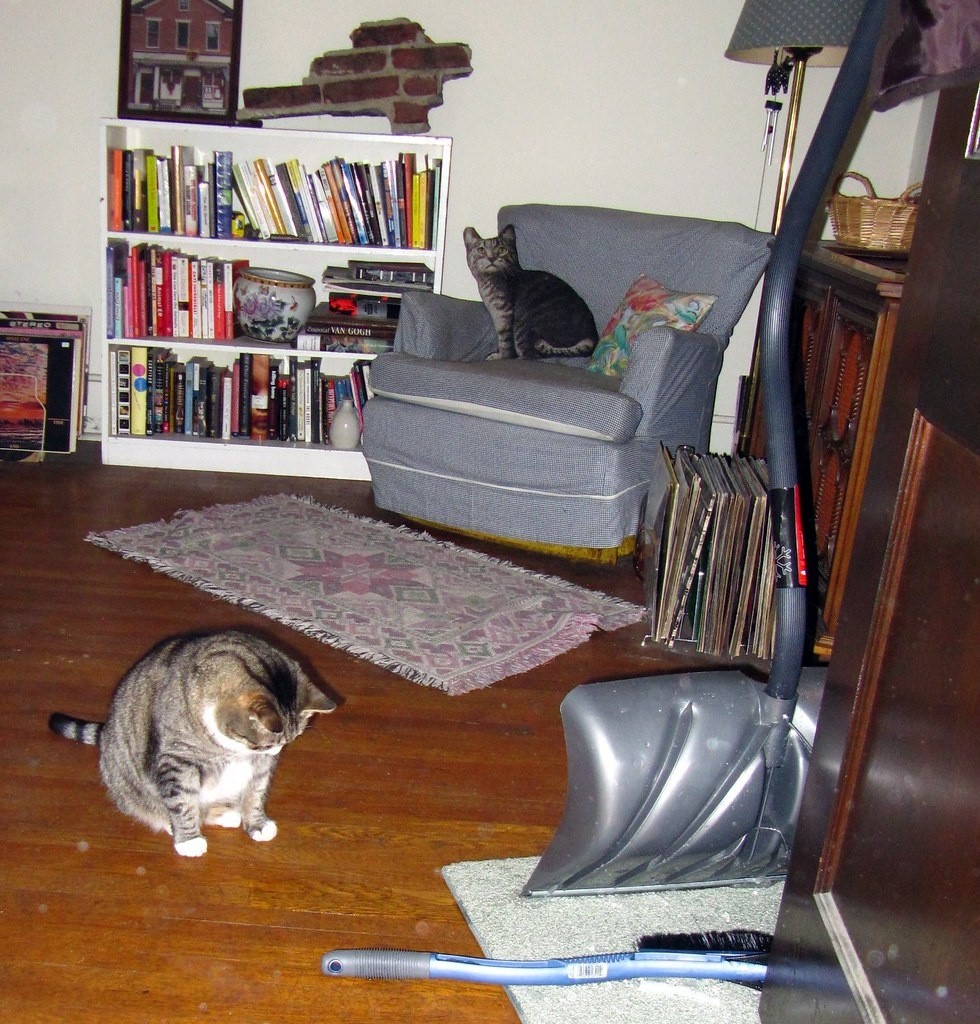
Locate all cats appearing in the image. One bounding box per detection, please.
[462,224,599,363]
[47,627,337,857]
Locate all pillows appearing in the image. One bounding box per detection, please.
[585,270,719,382]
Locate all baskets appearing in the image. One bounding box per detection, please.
[824,171,923,253]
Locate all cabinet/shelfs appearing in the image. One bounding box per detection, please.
[734,256,915,663]
[98,118,454,482]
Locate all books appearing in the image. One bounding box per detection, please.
[110,145,443,444]
[646,440,778,657]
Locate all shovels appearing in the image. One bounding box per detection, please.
[524,0,890,900]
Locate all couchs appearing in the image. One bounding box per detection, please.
[362,203,777,567]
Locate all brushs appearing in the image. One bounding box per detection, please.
[318,927,775,988]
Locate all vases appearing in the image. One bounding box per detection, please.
[329,398,360,450]
[233,266,315,341]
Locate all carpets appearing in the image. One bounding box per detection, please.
[81,489,652,698]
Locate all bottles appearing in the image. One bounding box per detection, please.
[328,398,362,451]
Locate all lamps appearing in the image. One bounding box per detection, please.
[722,0,867,456]
